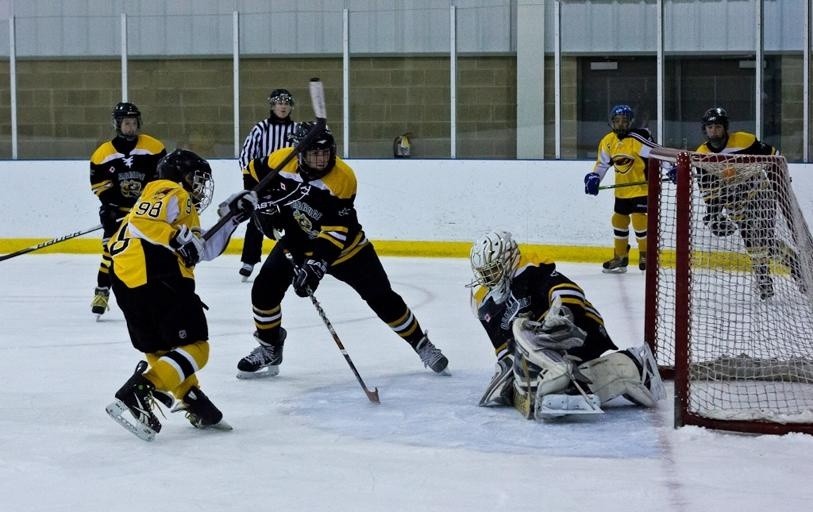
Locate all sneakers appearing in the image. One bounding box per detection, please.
[756,276,774,300]
[791,269,808,293]
[240,263,253,276]
[91,287,109,314]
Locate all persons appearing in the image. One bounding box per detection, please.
[107,148,258,433]
[89,102,167,316]
[237,120,450,374]
[237,89,301,277]
[583,105,656,271]
[693,107,808,301]
[468,229,667,420]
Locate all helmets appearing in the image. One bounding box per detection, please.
[112,102,141,138]
[701,108,729,141]
[608,105,634,134]
[266,89,295,106]
[293,121,337,176]
[154,149,214,215]
[465,232,521,305]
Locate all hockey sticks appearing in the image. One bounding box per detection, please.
[200,77,327,244]
[274,229,379,404]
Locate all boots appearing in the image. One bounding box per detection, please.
[238,327,286,372]
[116,360,174,433]
[171,386,222,429]
[639,252,647,270]
[603,245,630,269]
[416,337,448,373]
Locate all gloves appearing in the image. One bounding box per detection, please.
[666,166,677,184]
[703,214,734,236]
[99,205,116,231]
[218,190,285,240]
[585,173,599,195]
[170,224,204,268]
[294,259,327,297]
